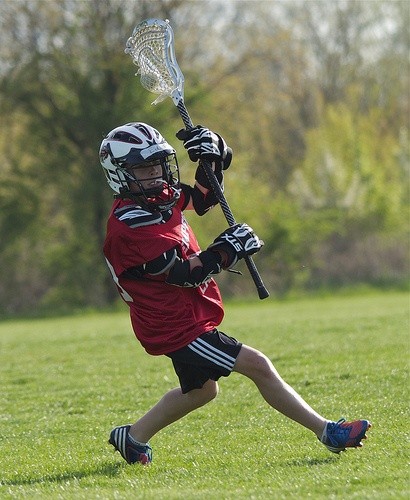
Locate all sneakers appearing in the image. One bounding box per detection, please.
[108,424,152,465]
[317,418,372,455]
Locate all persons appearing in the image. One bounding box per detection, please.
[97,121,370,469]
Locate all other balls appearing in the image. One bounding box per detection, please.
[140,72,158,90]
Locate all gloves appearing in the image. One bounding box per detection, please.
[208,222,264,270]
[176,124,232,170]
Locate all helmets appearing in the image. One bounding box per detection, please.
[99,122,176,197]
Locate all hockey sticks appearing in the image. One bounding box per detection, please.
[124,17,272,300]
